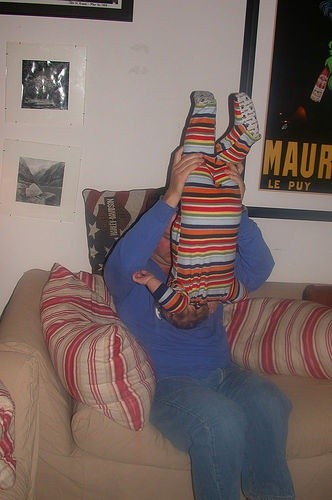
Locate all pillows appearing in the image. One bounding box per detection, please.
[223,297,332,380]
[76,270,117,314]
[40,263,156,432]
[81,188,157,276]
[0,380,16,490]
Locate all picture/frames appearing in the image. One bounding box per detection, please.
[0,0,134,23]
[239,0,332,222]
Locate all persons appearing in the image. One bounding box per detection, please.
[132,90,262,330]
[103,146,296,500]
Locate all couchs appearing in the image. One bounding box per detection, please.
[0,268,332,500]
